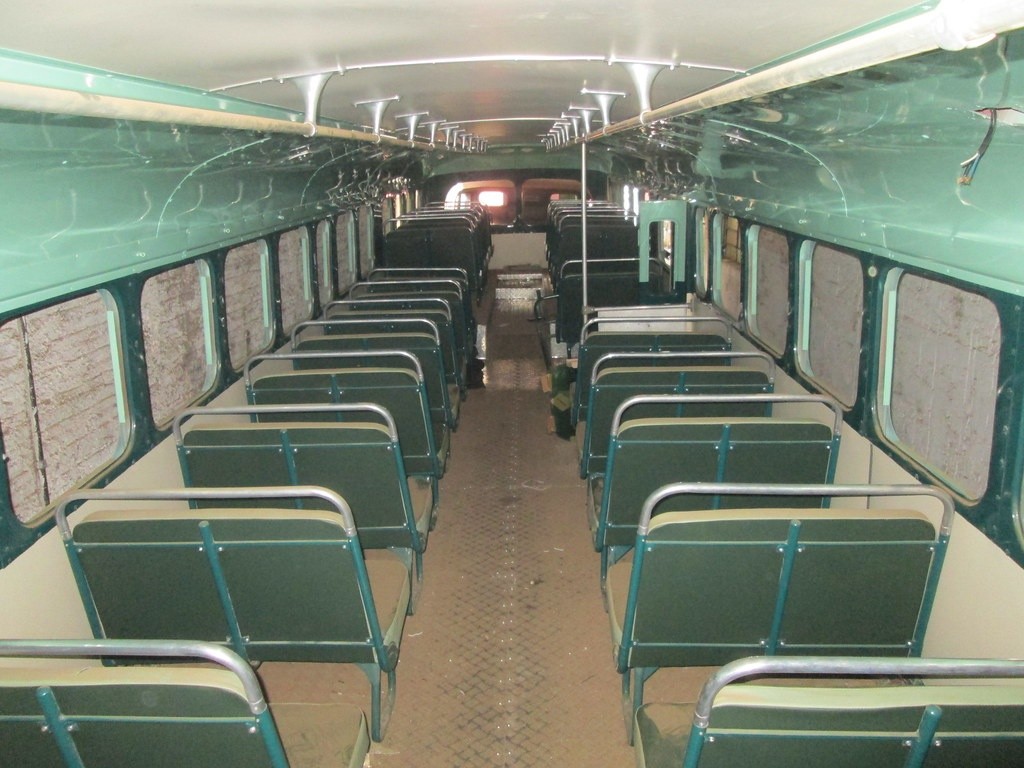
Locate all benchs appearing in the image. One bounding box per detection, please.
[0,200,1024,768]
